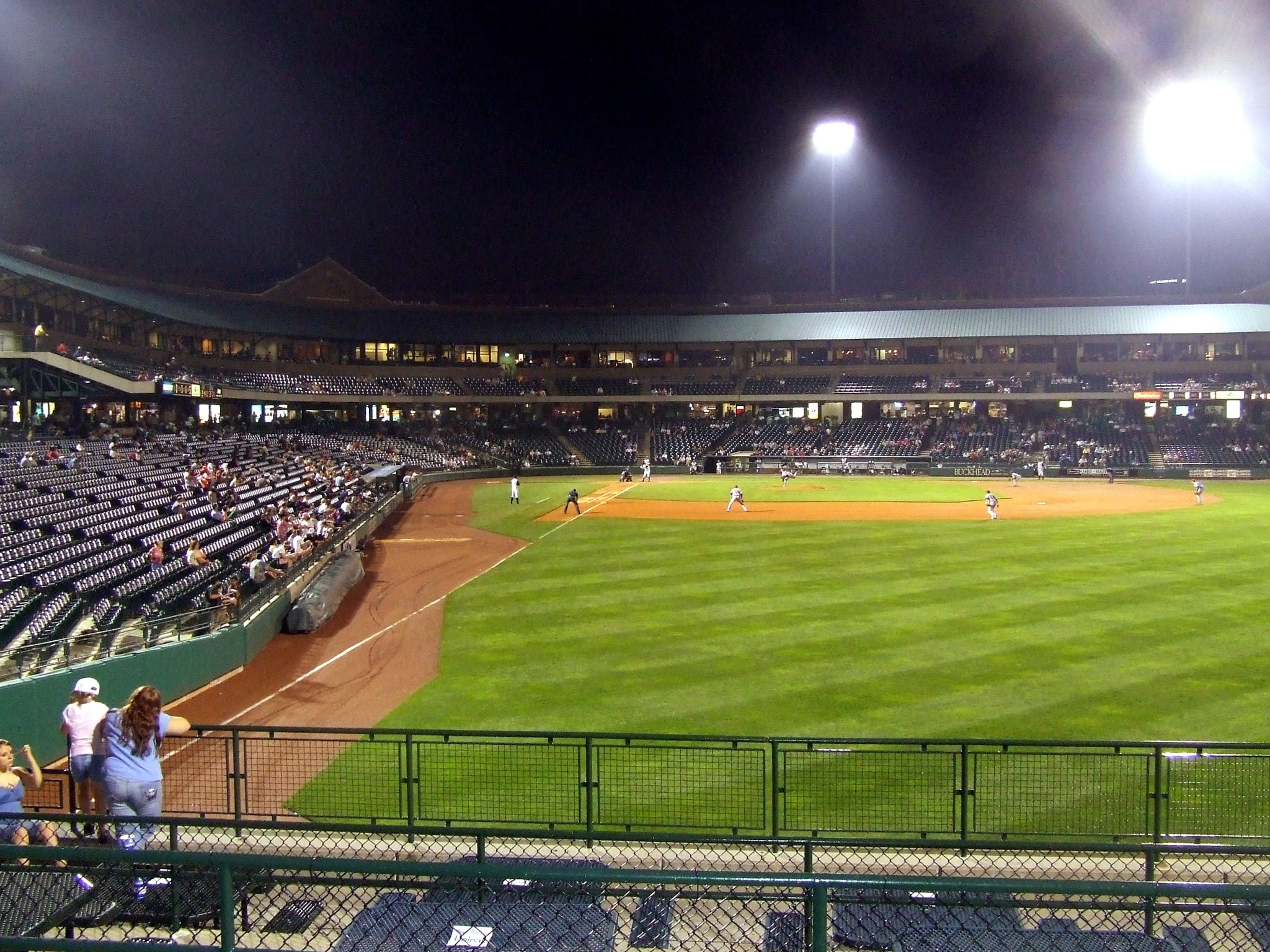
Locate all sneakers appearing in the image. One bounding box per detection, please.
[130,880,147,899]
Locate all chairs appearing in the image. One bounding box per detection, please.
[0,369,1270,670]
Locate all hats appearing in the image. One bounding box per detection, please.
[69,677,100,696]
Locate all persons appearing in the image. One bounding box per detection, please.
[530,388,534,395]
[467,357,472,362]
[726,486,747,512]
[511,475,520,504]
[0,320,580,636]
[539,388,546,396]
[101,686,191,898]
[0,738,67,866]
[558,351,1270,486]
[60,677,115,843]
[985,490,999,519]
[564,489,580,514]
[1191,479,1204,503]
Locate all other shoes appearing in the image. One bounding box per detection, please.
[97,829,114,844]
[83,822,96,835]
